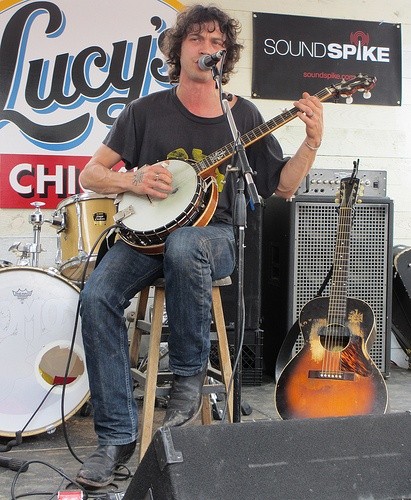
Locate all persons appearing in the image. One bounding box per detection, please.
[75,4,324,487]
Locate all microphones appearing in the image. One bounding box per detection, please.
[199,49,227,70]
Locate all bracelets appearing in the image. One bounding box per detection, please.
[304,142,318,150]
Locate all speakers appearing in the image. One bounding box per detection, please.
[282,195,394,379]
[202,191,262,330]
[121,412,411,500]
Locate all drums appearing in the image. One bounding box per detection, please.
[0,265,92,438]
[53,192,120,284]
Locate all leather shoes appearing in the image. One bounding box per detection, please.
[162,362,207,427]
[76,438,138,487]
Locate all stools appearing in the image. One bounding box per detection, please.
[129,276,233,465]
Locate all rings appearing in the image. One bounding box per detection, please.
[310,112,314,117]
[156,174,158,180]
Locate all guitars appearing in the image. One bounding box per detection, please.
[110,74,378,257]
[273,159,390,421]
